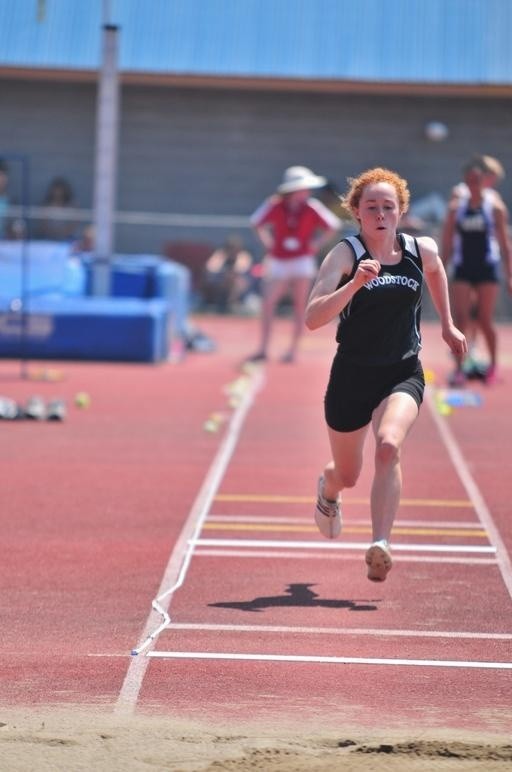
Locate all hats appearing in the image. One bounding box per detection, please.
[277,166,327,195]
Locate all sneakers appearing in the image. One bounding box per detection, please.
[314,473,342,539]
[365,539,393,582]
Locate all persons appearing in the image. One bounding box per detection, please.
[37,177,75,238]
[204,234,254,312]
[450,155,506,378]
[442,159,512,385]
[249,165,341,362]
[306,168,467,582]
[0,153,28,238]
